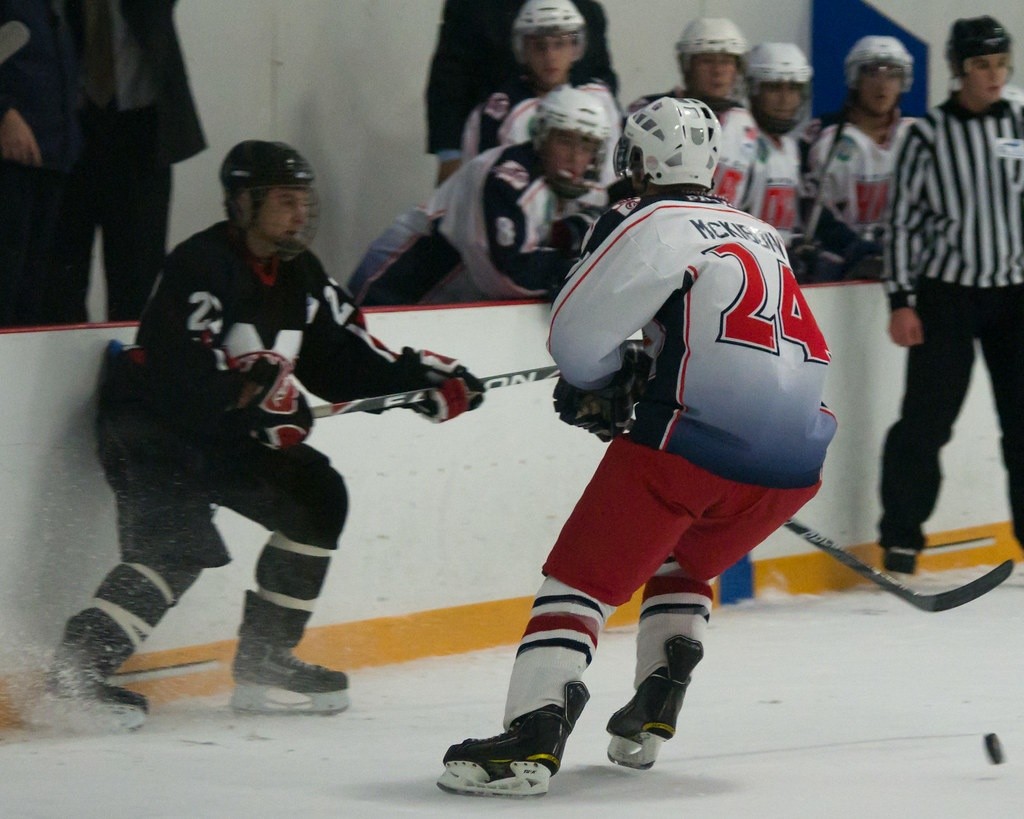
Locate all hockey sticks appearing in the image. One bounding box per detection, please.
[805,83,863,241]
[310,364,561,419]
[625,416,1015,614]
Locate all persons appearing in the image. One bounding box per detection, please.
[805,36,918,273]
[709,44,833,280]
[421,0,621,188]
[461,0,586,161]
[881,14,1024,575]
[0,0,207,331]
[443,98,838,774]
[343,86,612,308]
[622,16,747,122]
[42,139,487,711]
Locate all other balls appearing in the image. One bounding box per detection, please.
[984,732,1004,765]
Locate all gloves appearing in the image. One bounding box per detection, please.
[388,347,486,422]
[235,372,312,449]
[553,377,634,442]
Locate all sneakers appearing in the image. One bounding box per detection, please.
[436,680,591,795]
[228,588,354,714]
[29,616,147,727]
[882,547,918,581]
[606,635,704,770]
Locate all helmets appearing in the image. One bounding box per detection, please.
[221,140,315,217]
[948,16,1013,78]
[529,89,610,151]
[677,17,748,71]
[843,34,913,91]
[513,0,586,62]
[624,97,723,189]
[744,42,813,100]
